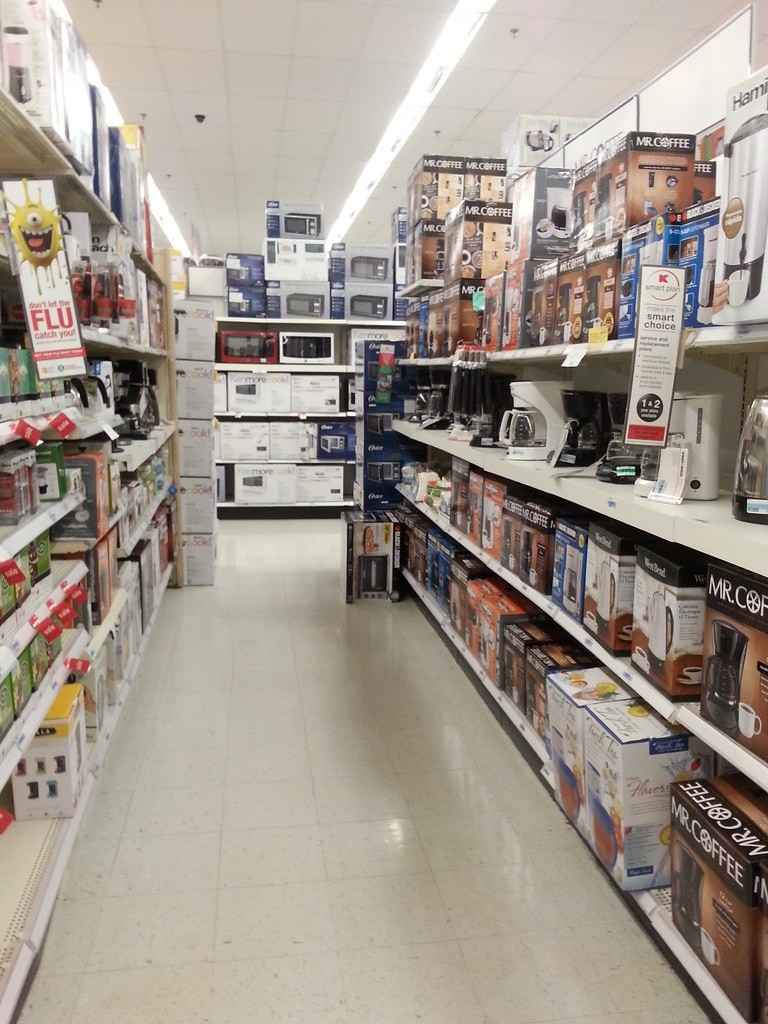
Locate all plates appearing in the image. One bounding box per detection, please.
[632,654,650,674]
[584,618,597,634]
[680,681,702,684]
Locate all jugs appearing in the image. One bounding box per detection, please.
[454,413,474,432]
[499,410,546,447]
[705,655,739,709]
[521,549,530,574]
[416,391,428,414]
[564,568,576,603]
[722,113,768,299]
[640,435,681,481]
[698,261,715,307]
[477,414,493,435]
[428,389,446,418]
[597,429,640,470]
[566,415,597,452]
[502,537,509,559]
[64,376,108,413]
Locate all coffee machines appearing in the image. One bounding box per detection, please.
[408,370,723,501]
[427,172,613,356]
[111,360,161,437]
[695,224,719,324]
[407,482,748,957]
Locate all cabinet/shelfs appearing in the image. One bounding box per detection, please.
[215,316,406,507]
[0,88,176,1024]
[393,323,768,1024]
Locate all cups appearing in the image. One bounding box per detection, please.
[739,702,762,739]
[593,318,602,328]
[701,927,721,966]
[482,530,487,548]
[623,626,632,635]
[530,569,538,585]
[533,710,539,729]
[540,327,547,345]
[635,646,650,672]
[683,667,703,681]
[586,611,598,630]
[605,217,614,239]
[513,687,518,703]
[509,554,516,569]
[564,322,574,342]
[727,270,750,306]
[457,512,461,525]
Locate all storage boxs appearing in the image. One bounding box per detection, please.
[0,0,406,823]
[518,501,577,596]
[395,504,439,591]
[406,66,768,359]
[699,562,768,763]
[475,596,541,691]
[449,456,478,535]
[629,544,712,704]
[449,558,496,641]
[481,477,521,560]
[503,621,568,715]
[670,774,768,1024]
[545,667,640,840]
[467,470,496,548]
[425,528,453,596]
[585,698,715,892]
[523,644,601,743]
[435,537,472,615]
[583,521,640,658]
[500,494,540,576]
[550,517,591,625]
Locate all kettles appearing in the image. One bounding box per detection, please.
[731,393,768,525]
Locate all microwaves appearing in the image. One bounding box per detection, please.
[228,213,388,319]
[323,412,400,482]
[221,331,334,364]
